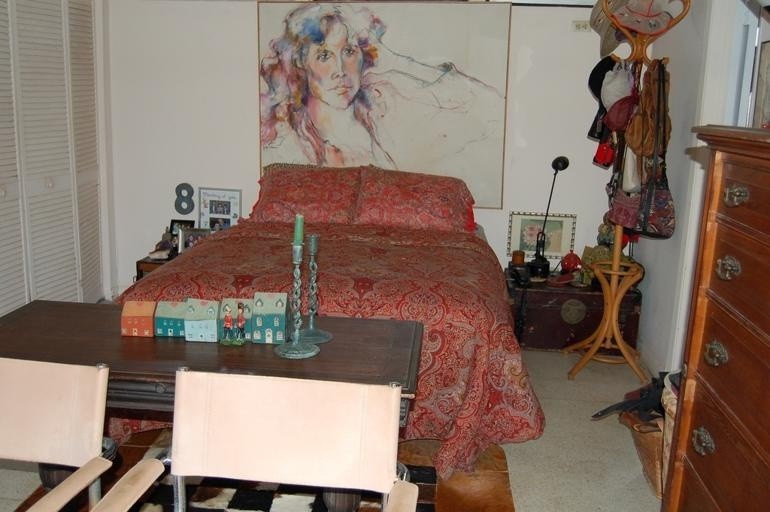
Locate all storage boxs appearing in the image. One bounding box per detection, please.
[506,263,642,355]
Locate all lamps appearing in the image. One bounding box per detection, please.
[524,156,569,281]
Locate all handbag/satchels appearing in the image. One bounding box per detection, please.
[588,60,676,245]
[631,424,663,502]
[582,245,626,270]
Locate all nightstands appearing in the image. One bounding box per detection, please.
[137,256,166,278]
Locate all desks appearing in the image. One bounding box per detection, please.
[1,299,423,512]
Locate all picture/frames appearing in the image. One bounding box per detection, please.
[507,211,577,259]
[198,187,242,234]
[169,220,195,237]
[179,228,210,256]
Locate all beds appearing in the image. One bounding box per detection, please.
[102,164,549,478]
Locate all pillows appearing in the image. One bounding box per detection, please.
[353,166,476,232]
[251,162,360,228]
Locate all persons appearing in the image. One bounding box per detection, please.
[211,202,229,215]
[220,304,235,341]
[212,219,227,232]
[235,302,249,339]
[171,222,189,235]
[186,233,203,249]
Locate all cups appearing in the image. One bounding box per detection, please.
[511,250,524,264]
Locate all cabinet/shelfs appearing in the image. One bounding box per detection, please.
[657,125,770,512]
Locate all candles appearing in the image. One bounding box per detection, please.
[294,213,304,246]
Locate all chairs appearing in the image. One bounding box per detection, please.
[0,358,112,511]
[93,369,420,511]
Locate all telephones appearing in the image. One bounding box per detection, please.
[509,262,529,288]
